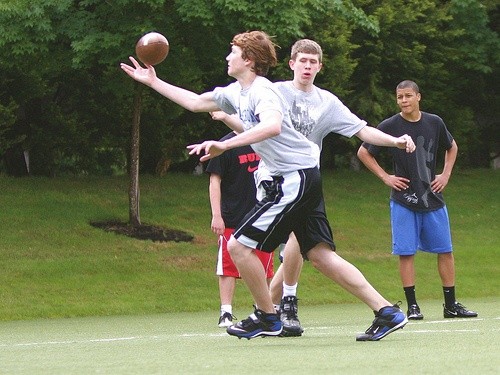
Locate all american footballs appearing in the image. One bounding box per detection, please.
[135,32,169,65]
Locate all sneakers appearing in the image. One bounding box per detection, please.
[408,303,423,320]
[226,309,282,341]
[444,302,478,318]
[281,295,301,337]
[219,312,233,327]
[356,300,408,341]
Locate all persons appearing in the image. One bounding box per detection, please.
[120,31,408,341]
[208,38,416,337]
[356,80,478,319]
[205,131,273,328]
